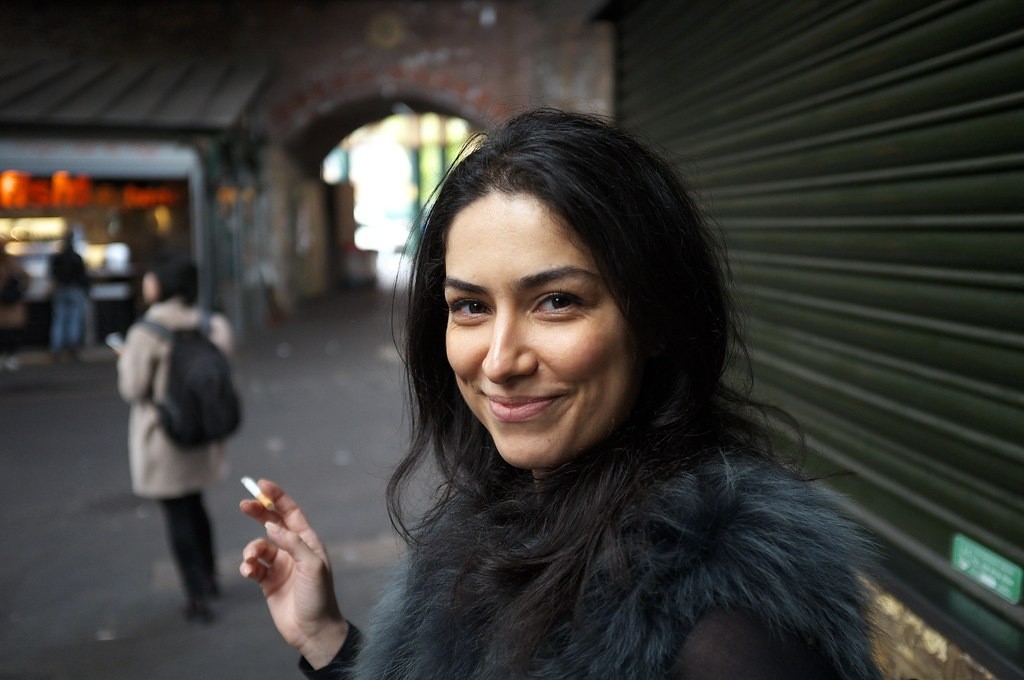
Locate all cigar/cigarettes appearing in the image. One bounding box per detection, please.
[241,475,274,512]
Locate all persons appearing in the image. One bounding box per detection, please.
[45,228,242,625]
[236,107,891,680]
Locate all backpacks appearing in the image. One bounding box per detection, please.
[134,309,240,449]
